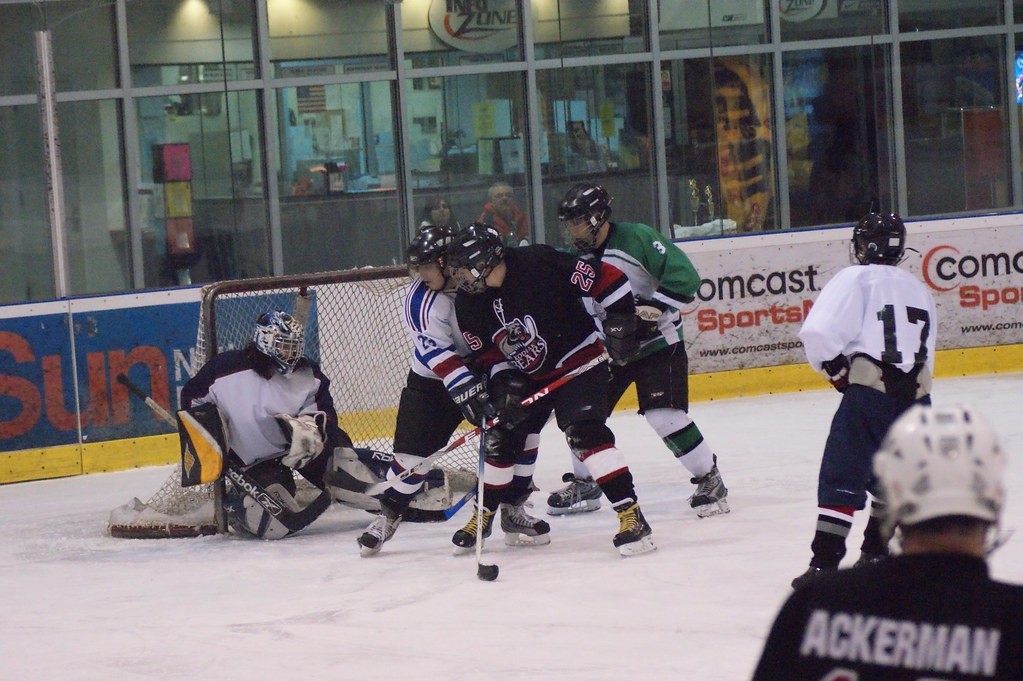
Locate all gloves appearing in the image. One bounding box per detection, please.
[822,353,850,396]
[450,378,495,427]
[634,286,685,341]
[601,313,639,365]
[488,369,535,433]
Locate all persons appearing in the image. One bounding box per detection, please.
[751,394,1022,681]
[180,307,445,541]
[358,220,653,548]
[477,181,529,247]
[791,210,936,590]
[547,180,727,508]
[418,193,461,234]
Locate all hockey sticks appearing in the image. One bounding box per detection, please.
[116,372,332,533]
[328,325,653,497]
[474,415,499,581]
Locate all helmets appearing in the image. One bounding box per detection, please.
[849,211,906,266]
[870,399,1005,526]
[443,222,505,294]
[559,183,614,251]
[406,225,458,279]
[250,311,304,375]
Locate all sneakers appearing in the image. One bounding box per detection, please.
[687,453,731,518]
[452,498,497,556]
[500,488,551,546]
[791,559,839,593]
[547,472,603,515]
[357,495,402,557]
[852,549,889,573]
[612,497,656,557]
